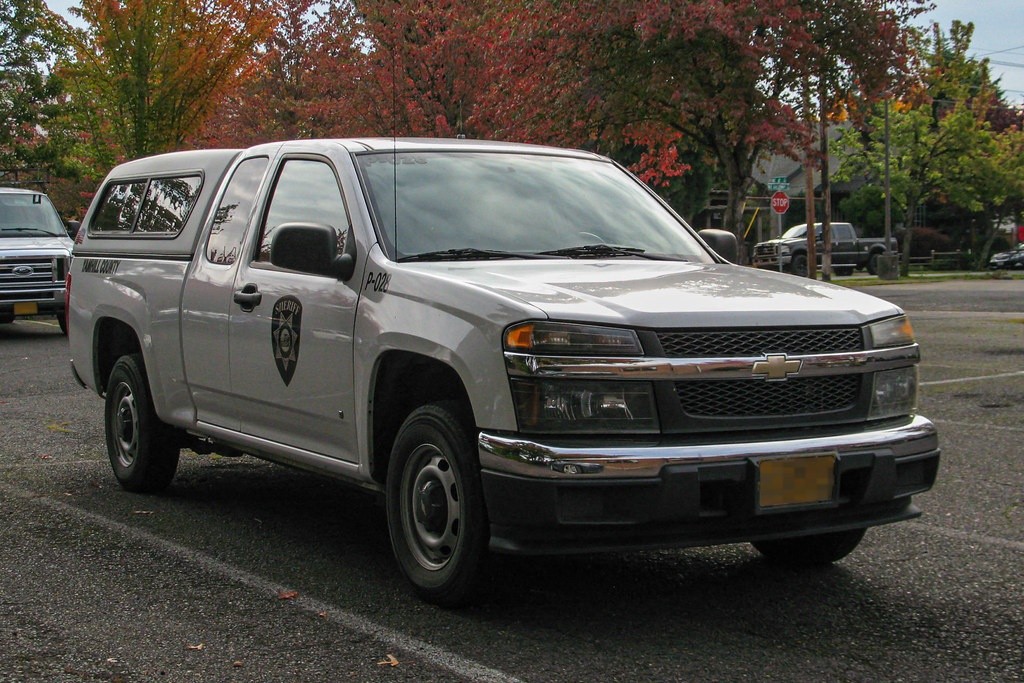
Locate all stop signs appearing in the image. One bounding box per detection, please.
[772,192,790,214]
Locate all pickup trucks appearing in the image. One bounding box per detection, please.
[64,132,933,605]
[752,221,898,281]
[0,185,83,337]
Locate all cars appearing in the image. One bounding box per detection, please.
[989,243,1024,270]
